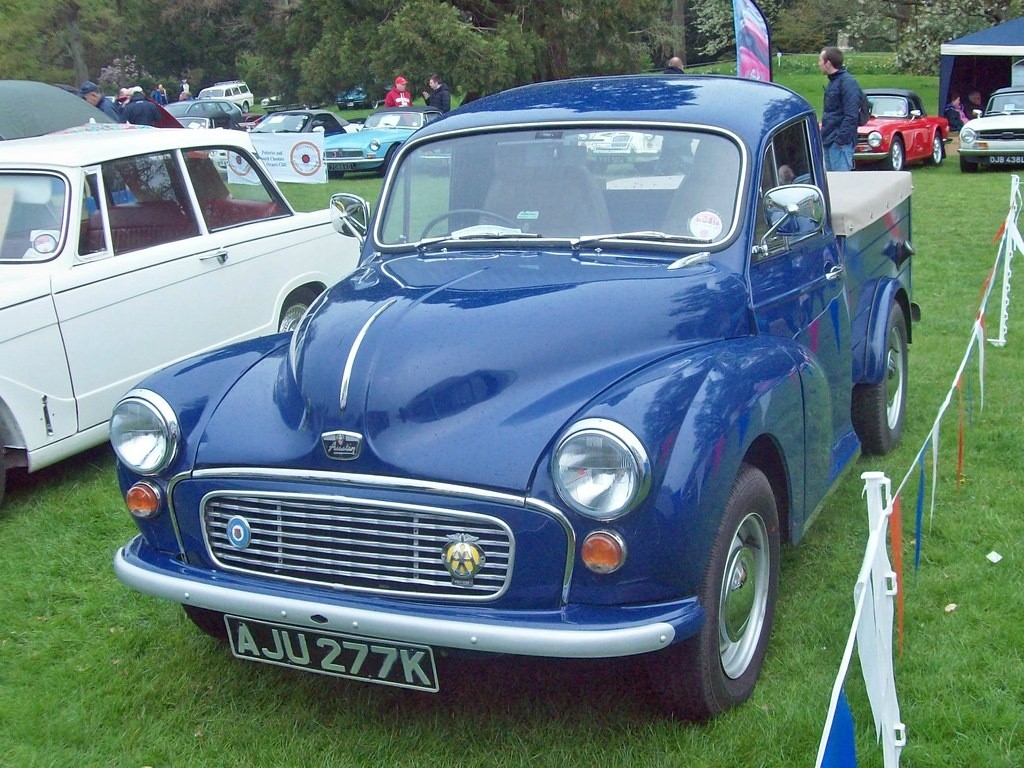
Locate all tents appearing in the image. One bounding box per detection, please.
[938,17,1024,117]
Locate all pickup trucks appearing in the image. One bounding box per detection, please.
[113,74,925,724]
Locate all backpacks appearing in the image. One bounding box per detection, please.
[838,75,873,126]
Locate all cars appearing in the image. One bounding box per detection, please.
[573,130,700,176]
[333,79,417,110]
[0,125,366,509]
[161,98,366,169]
[420,144,452,172]
[261,91,327,114]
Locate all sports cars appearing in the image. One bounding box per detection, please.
[319,106,442,175]
[956,85,1024,173]
[817,88,954,172]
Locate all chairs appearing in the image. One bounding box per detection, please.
[663,136,740,236]
[476,141,613,239]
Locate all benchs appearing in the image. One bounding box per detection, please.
[81,195,278,255]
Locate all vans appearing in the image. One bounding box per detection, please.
[196,81,256,114]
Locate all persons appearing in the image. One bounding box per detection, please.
[385,77,412,107]
[945,95,971,130]
[778,167,795,186]
[178,92,191,101]
[764,195,822,218]
[664,57,684,73]
[423,75,450,114]
[78,83,121,208]
[114,86,161,125]
[151,85,167,106]
[820,48,860,171]
[963,93,984,119]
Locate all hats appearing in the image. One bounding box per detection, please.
[395,77,407,85]
[79,81,96,93]
[133,86,143,94]
